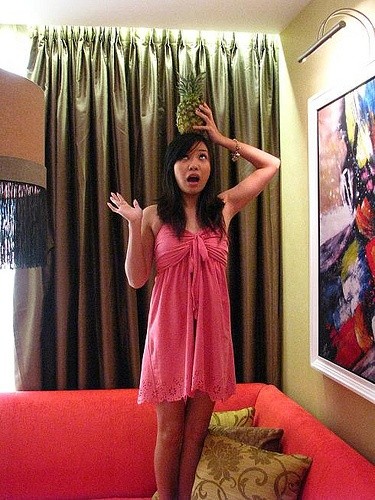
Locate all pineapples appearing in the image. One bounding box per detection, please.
[176,71,206,134]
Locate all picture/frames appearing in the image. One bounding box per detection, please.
[307,61,375,405]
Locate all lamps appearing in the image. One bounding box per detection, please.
[296,7,375,63]
[0,67,46,268]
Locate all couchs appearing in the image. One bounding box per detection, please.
[0,382,375,500]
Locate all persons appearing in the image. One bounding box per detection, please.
[108,103,281,500]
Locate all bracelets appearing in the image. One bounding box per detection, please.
[231,139,240,162]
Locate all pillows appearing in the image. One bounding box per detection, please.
[151,407,312,500]
